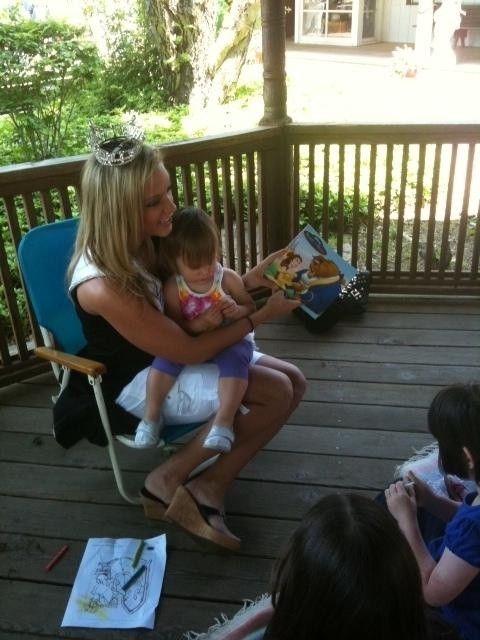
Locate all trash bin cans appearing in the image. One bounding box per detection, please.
[333,271,371,316]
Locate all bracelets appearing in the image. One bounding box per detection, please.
[245,316,255,333]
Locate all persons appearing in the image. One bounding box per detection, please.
[261,491,427,639]
[68,112,309,552]
[132,205,254,453]
[381,382,480,639]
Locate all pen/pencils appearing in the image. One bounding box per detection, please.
[122,565,146,590]
[45,544,69,571]
[404,481,415,487]
[132,539,146,568]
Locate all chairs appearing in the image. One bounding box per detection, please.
[18,216,251,504]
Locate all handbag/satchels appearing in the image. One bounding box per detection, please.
[339,271,369,314]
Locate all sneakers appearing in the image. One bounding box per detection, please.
[203,426,234,453]
[135,413,164,449]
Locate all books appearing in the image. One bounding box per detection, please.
[263,223,356,322]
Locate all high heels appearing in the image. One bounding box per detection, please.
[139,485,239,551]
